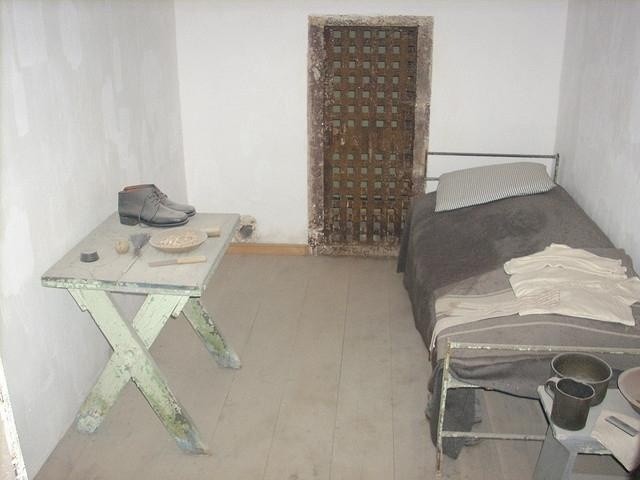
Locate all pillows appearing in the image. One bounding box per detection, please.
[434,161,555,212]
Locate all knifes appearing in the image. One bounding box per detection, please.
[147,255,207,267]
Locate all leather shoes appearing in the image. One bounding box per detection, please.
[124,184,196,217]
[118,188,190,226]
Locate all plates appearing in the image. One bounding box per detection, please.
[149,227,208,253]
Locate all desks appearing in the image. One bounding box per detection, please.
[40,209,244,456]
[534,384,639,477]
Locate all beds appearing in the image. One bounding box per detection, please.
[409,149,640,478]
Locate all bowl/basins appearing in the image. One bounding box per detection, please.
[550,354,613,407]
[618,365,640,415]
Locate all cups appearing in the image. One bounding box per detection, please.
[544,378,595,431]
